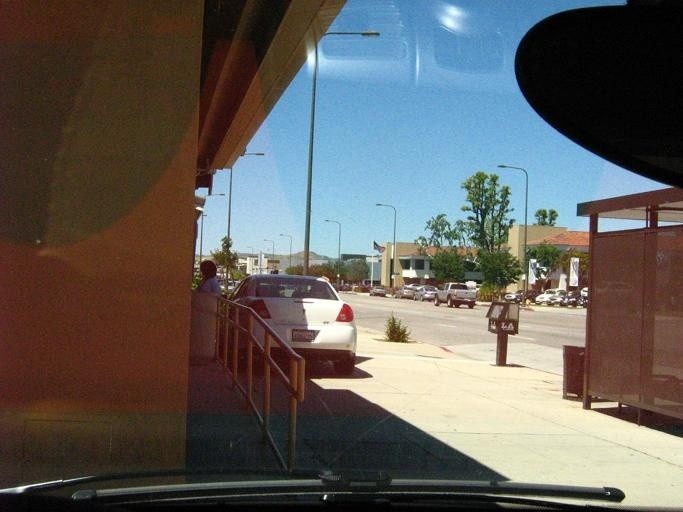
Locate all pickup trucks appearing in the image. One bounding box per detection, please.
[433,282,478,310]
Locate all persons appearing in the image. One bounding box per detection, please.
[197,260,221,296]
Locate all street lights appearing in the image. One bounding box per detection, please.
[197,192,226,286]
[279,233,294,275]
[324,219,341,291]
[495,164,529,306]
[228,233,277,274]
[224,151,265,295]
[375,203,398,288]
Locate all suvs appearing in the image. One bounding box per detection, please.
[504,287,588,308]
[594,279,636,298]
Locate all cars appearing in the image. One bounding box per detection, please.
[369,286,386,296]
[223,271,358,374]
[213,264,242,296]
[392,281,440,304]
